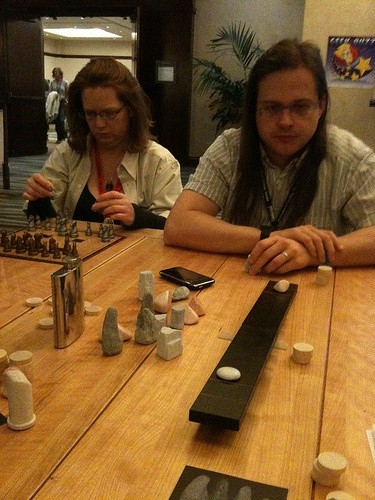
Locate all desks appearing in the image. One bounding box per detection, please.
[0,221,375,500]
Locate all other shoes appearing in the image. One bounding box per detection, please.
[55,139,65,144]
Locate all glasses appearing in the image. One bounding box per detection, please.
[77,104,125,121]
[255,96,321,122]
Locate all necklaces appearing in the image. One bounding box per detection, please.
[104,164,118,192]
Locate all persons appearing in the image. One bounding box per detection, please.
[163,38,375,277]
[42,67,69,144]
[24,58,183,230]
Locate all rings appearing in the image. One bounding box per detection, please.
[282,252,290,260]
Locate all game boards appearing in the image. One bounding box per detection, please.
[0,205,129,269]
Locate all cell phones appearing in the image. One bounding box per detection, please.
[159,266,215,291]
